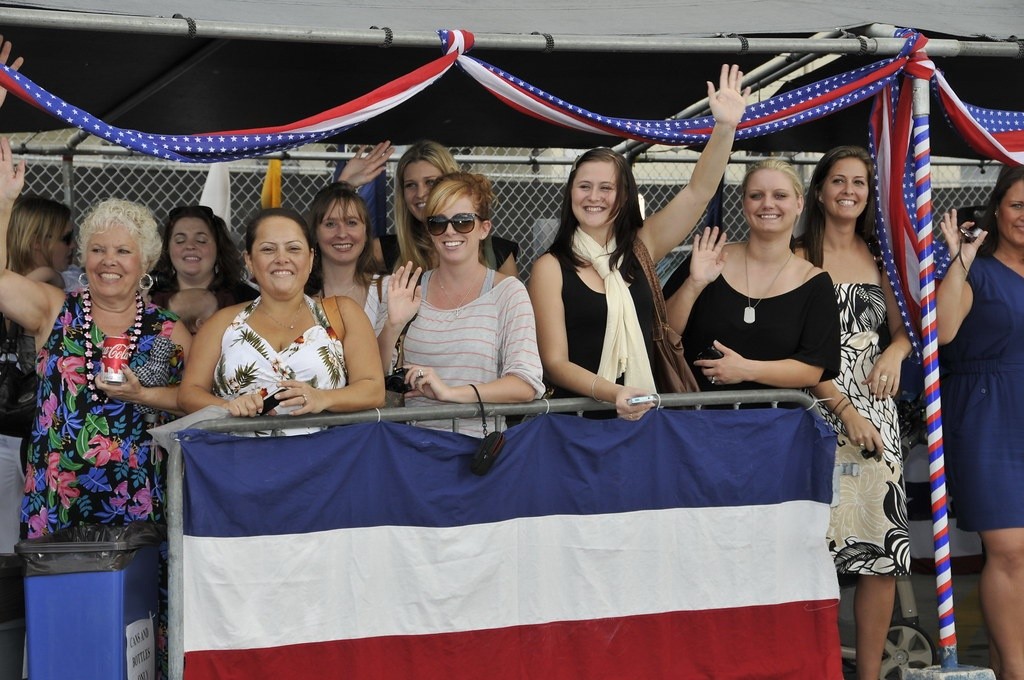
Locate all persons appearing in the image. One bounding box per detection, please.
[304,180,392,339]
[790,145,922,680]
[380,177,545,437]
[337,141,518,280]
[936,165,1024,680]
[144,205,262,335]
[530,65,752,421]
[0,32,79,553]
[662,158,841,410]
[177,207,386,416]
[0,136,191,541]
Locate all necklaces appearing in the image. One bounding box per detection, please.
[744,240,793,323]
[81,285,144,404]
[438,265,483,317]
[260,299,304,328]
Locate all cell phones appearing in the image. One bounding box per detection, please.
[628,396,654,406]
[697,347,724,369]
[256,386,289,417]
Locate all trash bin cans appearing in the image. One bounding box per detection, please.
[14,525,162,680]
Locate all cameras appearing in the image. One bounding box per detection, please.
[101,372,128,387]
[861,446,882,462]
[384,368,417,393]
[959,226,985,246]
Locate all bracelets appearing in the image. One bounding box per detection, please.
[838,402,852,417]
[591,375,602,402]
[832,397,846,412]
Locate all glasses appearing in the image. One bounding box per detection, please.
[169,205,215,221]
[426,212,485,236]
[37,229,75,247]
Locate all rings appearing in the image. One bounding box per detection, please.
[860,443,864,448]
[303,394,307,404]
[711,375,714,384]
[628,413,633,419]
[419,369,423,377]
[879,375,887,382]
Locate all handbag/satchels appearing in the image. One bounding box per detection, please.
[631,237,702,410]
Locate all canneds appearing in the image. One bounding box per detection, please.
[100,336,130,385]
